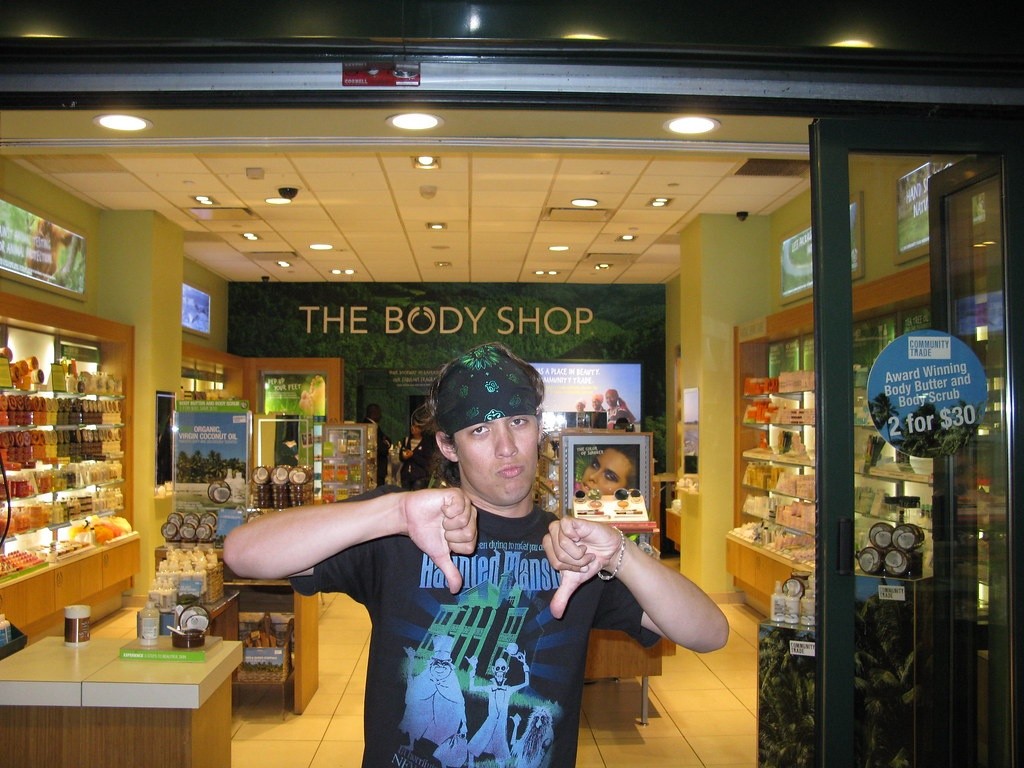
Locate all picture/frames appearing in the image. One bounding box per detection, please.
[559,430,653,556]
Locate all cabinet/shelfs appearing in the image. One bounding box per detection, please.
[0,630,244,768]
[322,420,381,504]
[1,384,140,650]
[540,430,561,519]
[154,545,321,715]
[732,385,960,624]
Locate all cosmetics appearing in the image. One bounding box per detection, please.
[208,480,231,503]
[321,438,375,502]
[223,469,234,500]
[139,511,223,646]
[0,614,13,649]
[734,369,931,628]
[250,465,313,507]
[572,488,644,514]
[0,347,130,580]
[233,472,245,503]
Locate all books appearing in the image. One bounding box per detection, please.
[120,636,205,662]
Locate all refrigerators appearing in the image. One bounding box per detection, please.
[322,422,377,504]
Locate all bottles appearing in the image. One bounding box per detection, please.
[0,613,12,647]
[147,546,218,613]
[783,582,801,624]
[771,580,784,623]
[322,463,362,503]
[799,589,815,628]
[0,459,123,538]
[139,601,160,647]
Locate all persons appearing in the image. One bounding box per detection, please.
[222,342,730,768]
[576,389,634,425]
[299,375,325,416]
[582,446,635,495]
[364,405,391,484]
[399,421,429,489]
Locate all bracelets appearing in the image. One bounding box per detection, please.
[597,527,625,580]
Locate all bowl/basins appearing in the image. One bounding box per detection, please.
[171,629,206,648]
